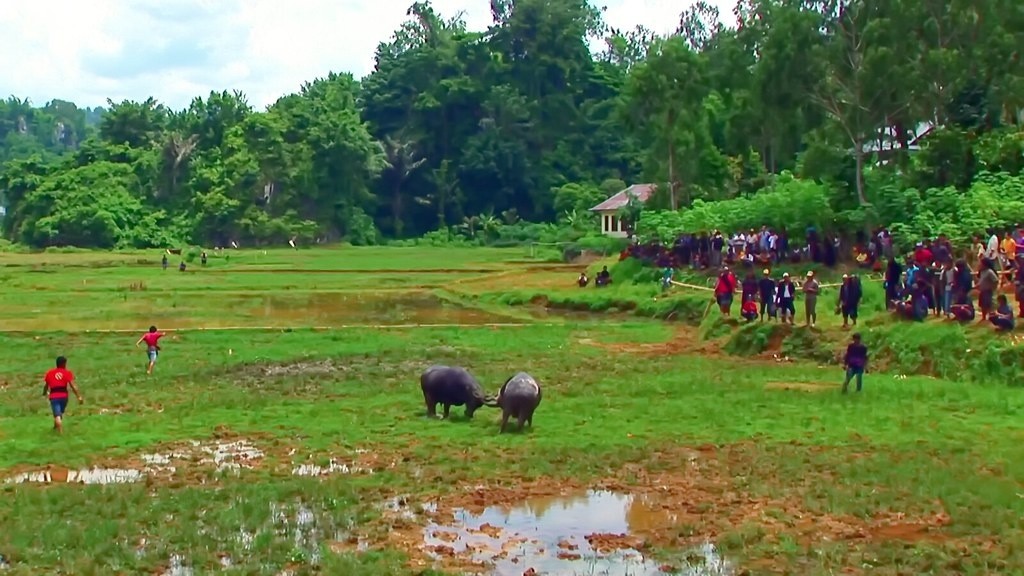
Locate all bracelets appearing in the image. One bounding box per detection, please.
[990,251,993,254]
[78,396,82,401]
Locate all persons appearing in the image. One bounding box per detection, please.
[715,267,863,328]
[135,326,166,375]
[579,266,609,287]
[42,356,83,433]
[840,334,868,392]
[660,264,674,288]
[884,222,1024,330]
[162,252,207,271]
[619,223,894,271]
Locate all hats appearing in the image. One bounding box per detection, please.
[807,270,814,276]
[723,267,728,272]
[782,272,788,277]
[762,269,769,274]
[916,242,922,249]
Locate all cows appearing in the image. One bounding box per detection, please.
[420,365,542,436]
